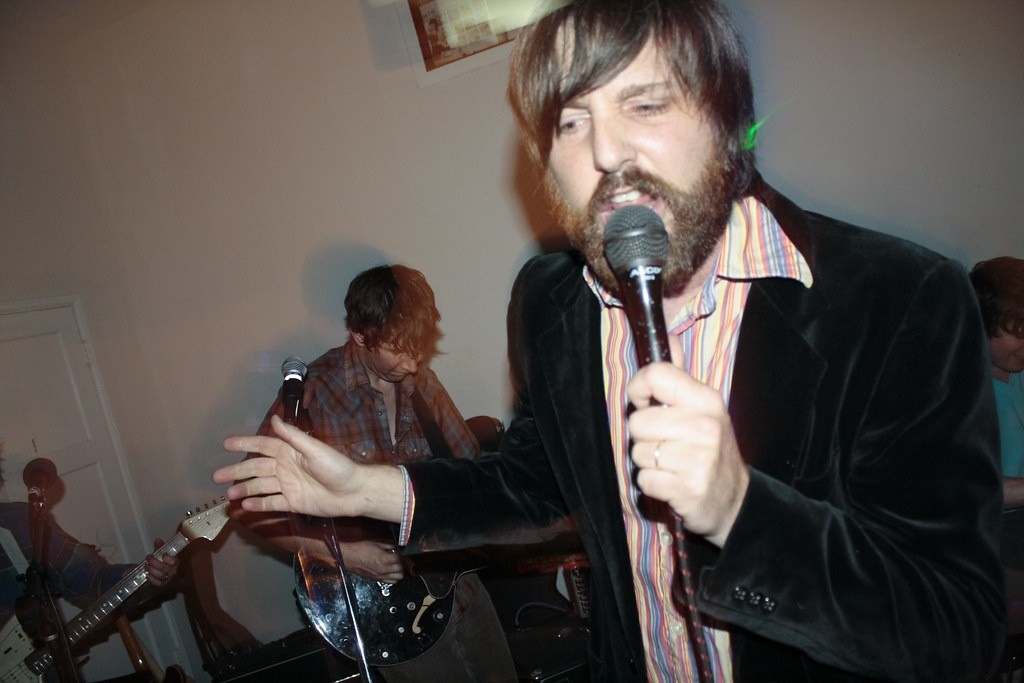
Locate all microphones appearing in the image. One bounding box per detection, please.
[280,356,308,426]
[22,457,58,504]
[600,201,671,408]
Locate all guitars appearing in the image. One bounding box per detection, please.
[287,515,500,672]
[0,493,233,683]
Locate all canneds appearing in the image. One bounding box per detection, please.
[562,556,593,619]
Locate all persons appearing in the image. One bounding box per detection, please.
[969,257,1024,671]
[0,442,179,683]
[212,0,1003,683]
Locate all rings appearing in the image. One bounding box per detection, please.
[654,440,665,470]
[160,574,170,581]
